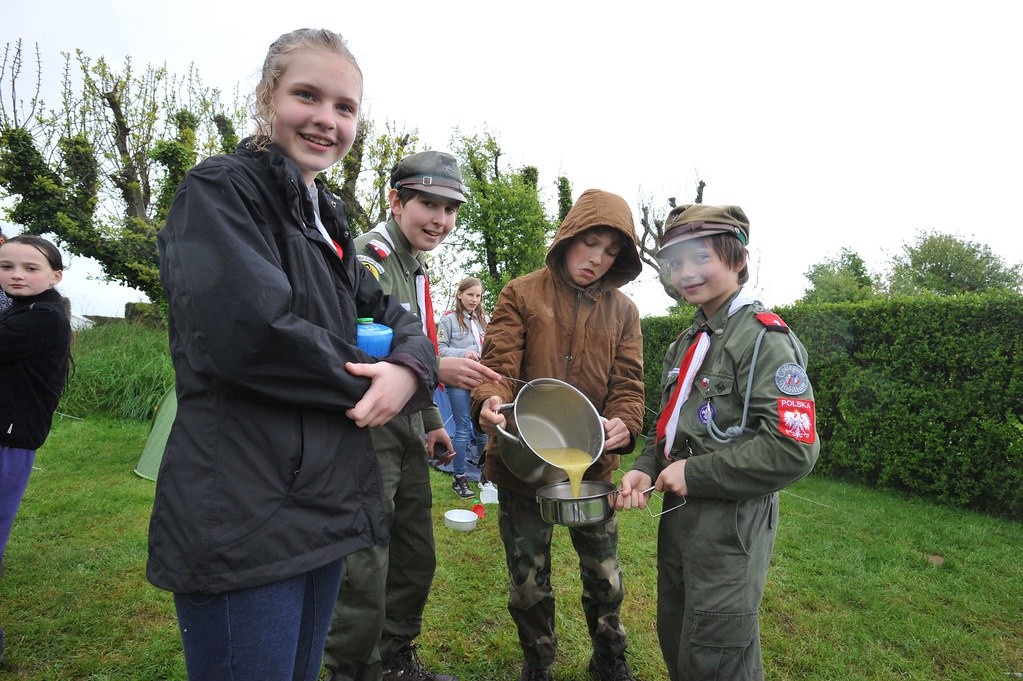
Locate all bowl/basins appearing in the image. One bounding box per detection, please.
[444,509,478,532]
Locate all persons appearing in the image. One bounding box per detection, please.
[0,230,73,563]
[436,277,497,499]
[323,150,468,681]
[145,28,441,681]
[469,189,646,681]
[614,204,821,681]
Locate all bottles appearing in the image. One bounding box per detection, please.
[355,318,394,359]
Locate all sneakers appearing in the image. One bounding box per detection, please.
[380,644,454,680]
[588,649,631,681]
[522,665,551,681]
[478,475,495,491]
[451,473,476,499]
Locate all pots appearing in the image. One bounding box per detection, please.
[535,481,687,528]
[494,378,611,487]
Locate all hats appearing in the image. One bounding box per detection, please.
[656,204,751,260]
[391,151,466,202]
